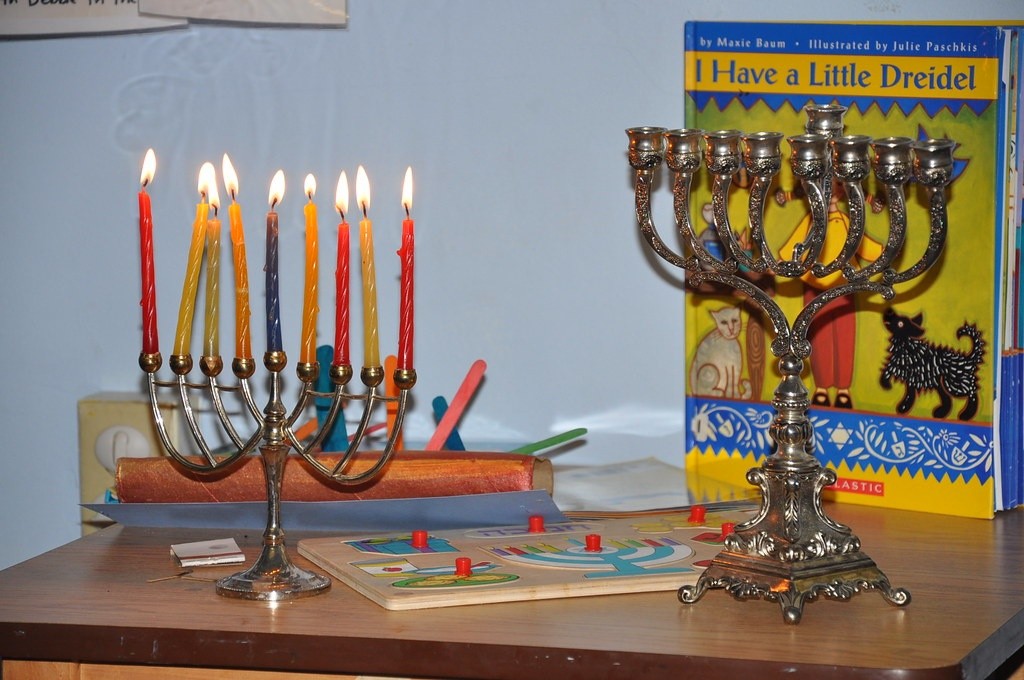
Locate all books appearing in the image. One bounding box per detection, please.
[171,538,246,569]
[680,18,1024,519]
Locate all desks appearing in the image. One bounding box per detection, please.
[0,488,1024,680]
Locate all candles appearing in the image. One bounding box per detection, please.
[300,204,318,362]
[174,204,209,356]
[395,220,413,371]
[138,191,160,355]
[359,219,380,369]
[334,222,350,365]
[229,204,251,359]
[266,212,283,351]
[202,219,222,358]
